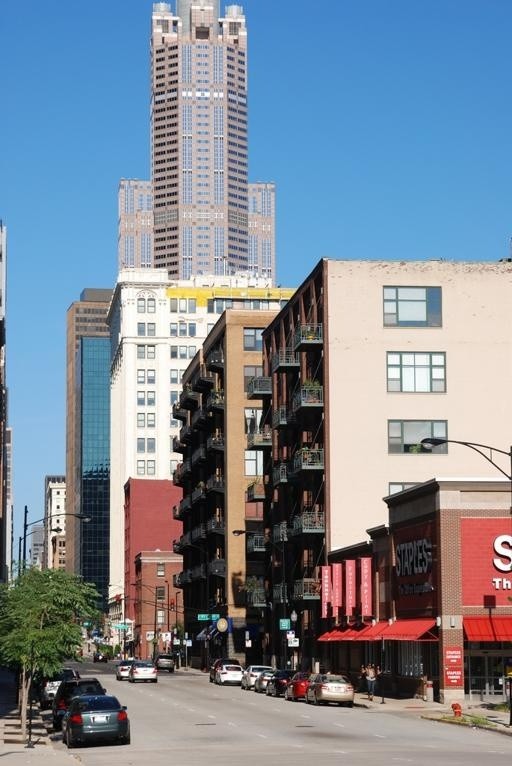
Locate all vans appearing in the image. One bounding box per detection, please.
[210,658,240,682]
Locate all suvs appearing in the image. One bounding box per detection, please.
[48,678,107,731]
[40,668,80,710]
[154,654,175,673]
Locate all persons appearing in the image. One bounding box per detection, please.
[354,663,383,701]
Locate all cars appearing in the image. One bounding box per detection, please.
[240,665,273,691]
[265,670,296,698]
[214,665,245,687]
[254,671,276,695]
[304,672,356,708]
[128,661,157,684]
[116,660,135,681]
[284,672,318,703]
[94,653,108,663]
[62,693,131,749]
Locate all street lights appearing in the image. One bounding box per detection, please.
[231,528,289,669]
[164,580,170,630]
[131,583,158,660]
[19,528,63,576]
[106,584,128,662]
[419,437,512,497]
[175,592,182,627]
[179,544,209,673]
[23,504,93,576]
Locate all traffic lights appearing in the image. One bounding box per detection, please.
[170,598,174,611]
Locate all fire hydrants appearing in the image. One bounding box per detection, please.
[452,703,462,716]
